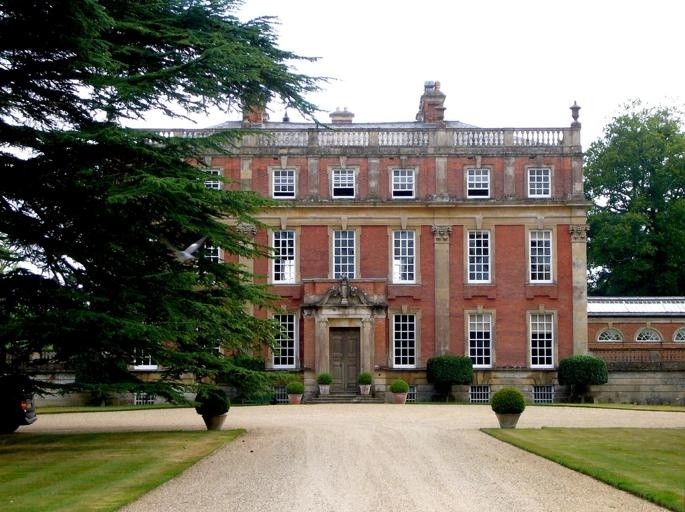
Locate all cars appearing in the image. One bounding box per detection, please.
[0,375,38,433]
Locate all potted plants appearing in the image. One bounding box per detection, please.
[194,388,230,431]
[288,381,304,405]
[388,380,410,404]
[317,373,333,394]
[358,372,372,394]
[491,386,527,429]
